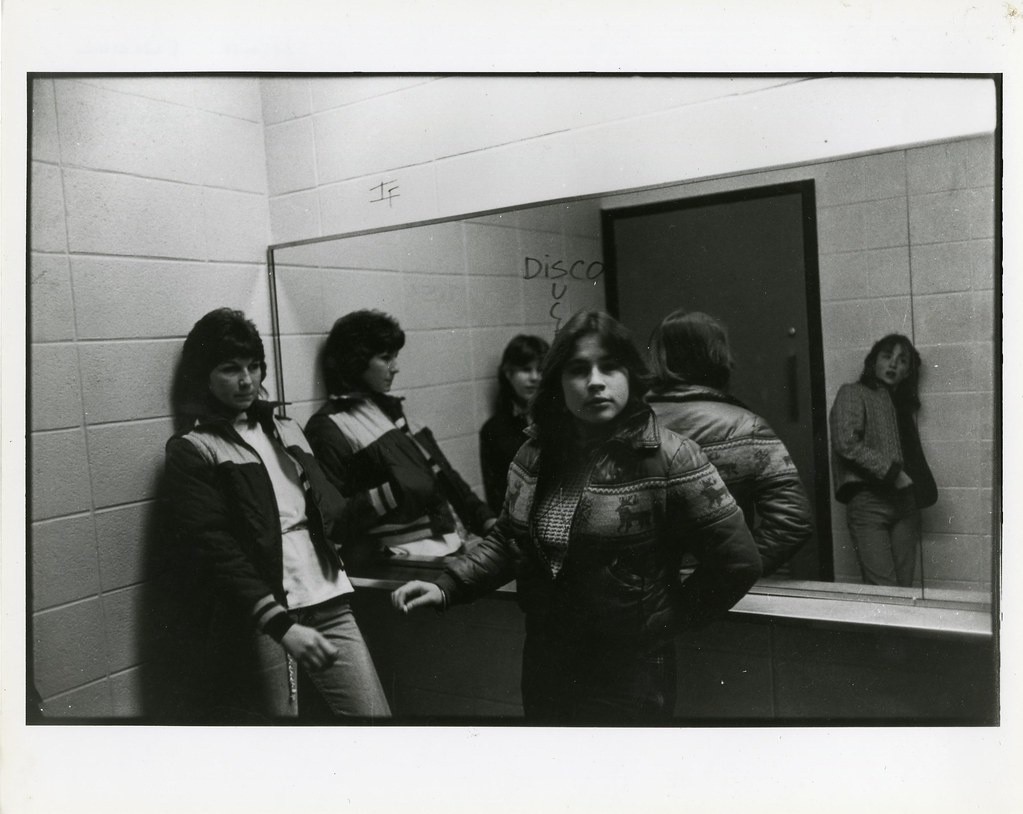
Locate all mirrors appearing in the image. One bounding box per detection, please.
[265,131,995,613]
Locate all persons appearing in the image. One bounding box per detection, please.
[167,309,392,726]
[642,307,815,578]
[829,334,939,586]
[390,309,764,727]
[303,308,501,570]
[480,335,549,516]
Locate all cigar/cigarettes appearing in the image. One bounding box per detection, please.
[334,660,352,666]
[403,604,408,613]
